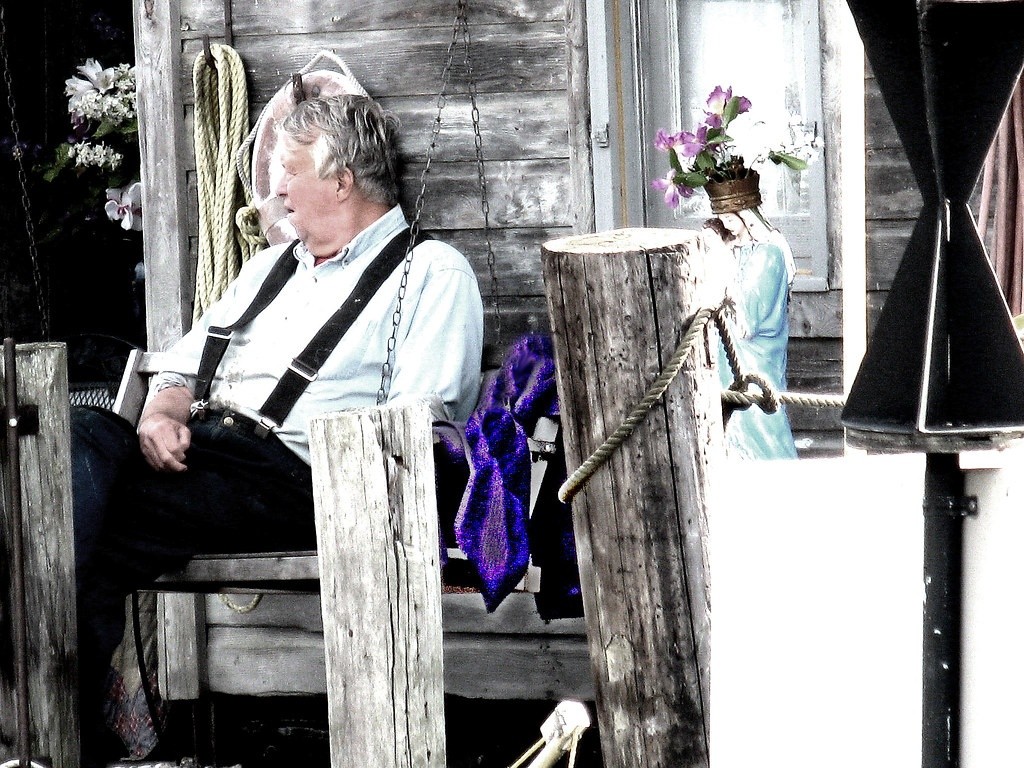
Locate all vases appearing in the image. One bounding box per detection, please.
[703,166,763,214]
[59,203,140,350]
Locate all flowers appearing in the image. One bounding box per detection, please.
[646,76,812,207]
[41,52,155,236]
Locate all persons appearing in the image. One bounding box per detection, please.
[69,92,485,768]
